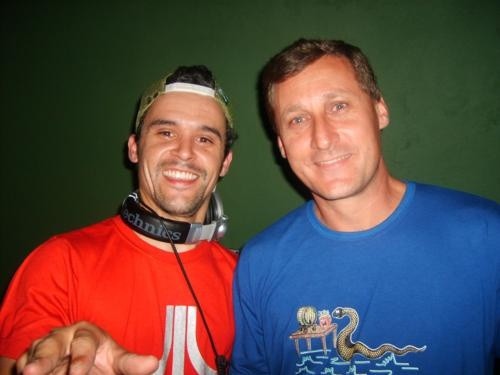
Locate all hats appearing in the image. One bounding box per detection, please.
[134,71,236,132]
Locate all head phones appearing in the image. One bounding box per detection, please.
[121,187,228,243]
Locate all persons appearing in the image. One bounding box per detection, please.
[228,37,500,375]
[0,65,240,375]
